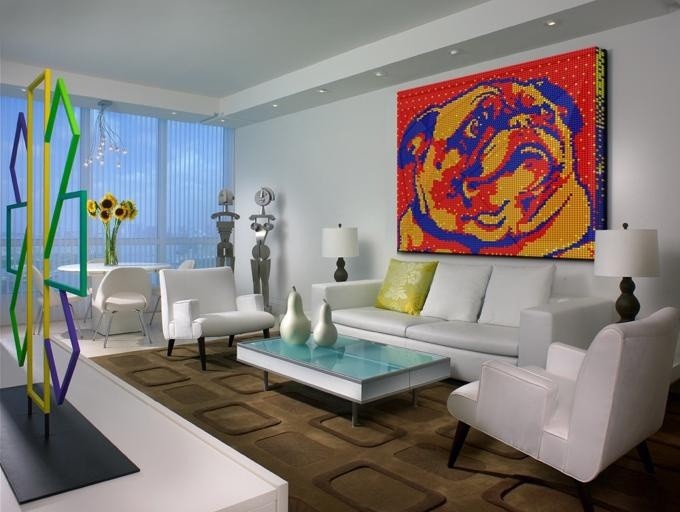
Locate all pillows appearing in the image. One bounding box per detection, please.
[477,264,557,326]
[419,261,492,323]
[376,258,439,317]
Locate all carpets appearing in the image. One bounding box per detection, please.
[90,331,680,512]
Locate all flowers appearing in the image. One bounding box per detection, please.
[88,193,138,265]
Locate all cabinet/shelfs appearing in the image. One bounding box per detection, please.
[0,333,288,511]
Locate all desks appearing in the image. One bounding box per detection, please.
[59,262,172,336]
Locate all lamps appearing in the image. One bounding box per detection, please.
[322,222,359,282]
[594,223,660,322]
[82,102,127,168]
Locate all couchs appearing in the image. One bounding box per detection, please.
[311,259,611,384]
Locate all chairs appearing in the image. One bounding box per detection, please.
[28,264,89,337]
[150,259,196,324]
[89,267,152,347]
[158,266,275,372]
[83,259,105,322]
[443,305,677,508]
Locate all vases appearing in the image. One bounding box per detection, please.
[105,232,118,265]
